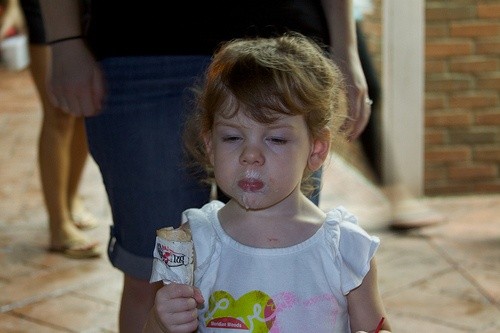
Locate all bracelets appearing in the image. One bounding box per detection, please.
[46,35,82,45]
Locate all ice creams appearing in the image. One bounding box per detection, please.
[156,228,191,242]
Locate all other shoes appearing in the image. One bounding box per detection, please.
[69,216,95,230]
[50,232,100,257]
[390,209,442,230]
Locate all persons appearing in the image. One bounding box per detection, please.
[41,0,371,333]
[142,37,392,333]
[17,0,101,258]
